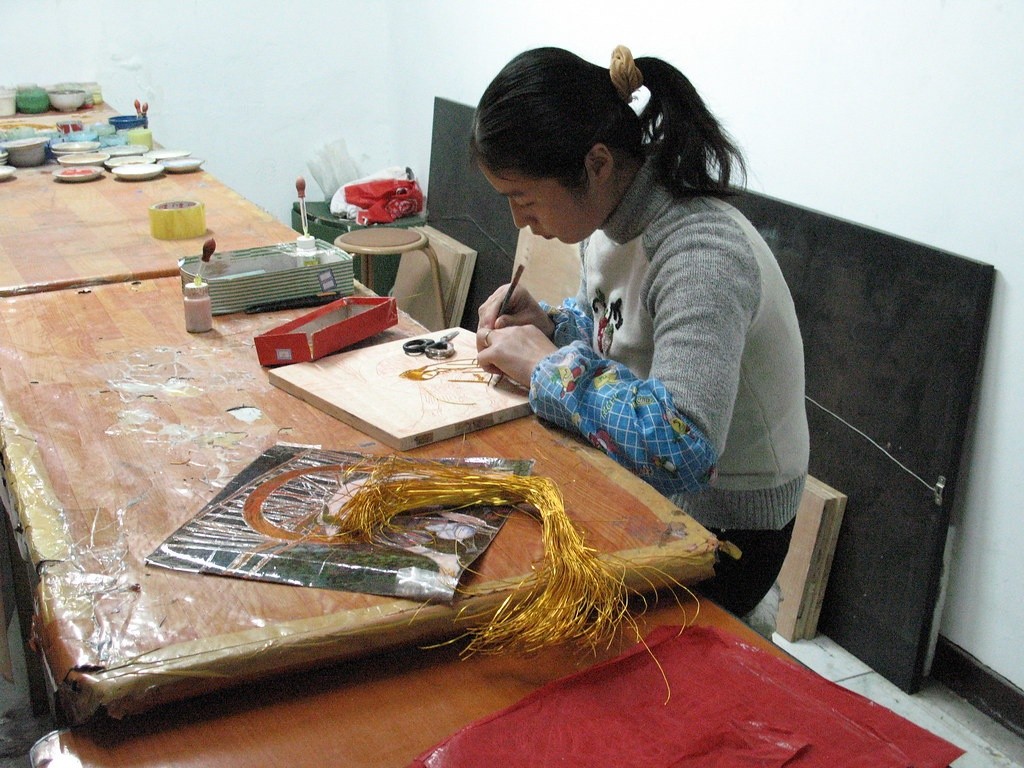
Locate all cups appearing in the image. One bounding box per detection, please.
[108,115,149,128]
[90,124,116,134]
[127,130,153,149]
[0,89,15,117]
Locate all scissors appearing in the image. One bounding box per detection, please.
[403,331,459,358]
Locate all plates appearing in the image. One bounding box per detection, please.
[143,148,190,159]
[0,165,17,181]
[0,151,9,165]
[58,153,110,166]
[112,164,164,180]
[157,157,205,171]
[104,156,155,169]
[97,145,149,156]
[54,166,105,181]
[52,141,101,159]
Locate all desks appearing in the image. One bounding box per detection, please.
[59,592,956,767]
[0,86,304,291]
[0,279,720,732]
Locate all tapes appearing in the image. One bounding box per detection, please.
[148,199,207,241]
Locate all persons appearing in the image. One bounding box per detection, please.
[469,47,810,621]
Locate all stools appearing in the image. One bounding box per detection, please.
[334,226,447,332]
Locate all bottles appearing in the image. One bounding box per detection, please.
[183,283,212,333]
[297,236,320,267]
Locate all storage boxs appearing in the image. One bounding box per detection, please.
[175,240,356,317]
[254,297,399,368]
[291,197,426,296]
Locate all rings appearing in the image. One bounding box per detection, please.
[484,331,491,347]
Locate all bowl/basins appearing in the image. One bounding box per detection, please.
[16,91,51,113]
[49,90,84,112]
[99,135,128,146]
[0,137,51,168]
[66,132,100,141]
